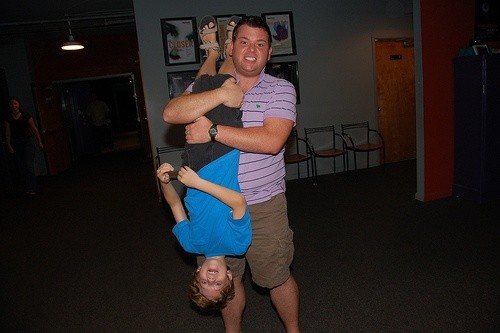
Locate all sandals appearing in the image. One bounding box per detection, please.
[223,16,241,58]
[198,15,220,62]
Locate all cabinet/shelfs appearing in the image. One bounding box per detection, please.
[451,52,500,205]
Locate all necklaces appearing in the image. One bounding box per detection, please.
[12,112,20,117]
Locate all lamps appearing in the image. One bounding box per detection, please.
[59,21,85,51]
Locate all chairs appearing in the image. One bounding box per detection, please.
[285,129,316,185]
[157,144,186,212]
[302,124,351,185]
[340,121,387,176]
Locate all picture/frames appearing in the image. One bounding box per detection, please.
[215,14,246,62]
[160,16,202,66]
[266,61,301,105]
[261,11,298,57]
[166,69,198,101]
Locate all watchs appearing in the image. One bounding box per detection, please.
[209,123,218,143]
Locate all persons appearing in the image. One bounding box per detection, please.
[163,17,300,333]
[4,96,43,196]
[83,91,112,155]
[156,16,252,309]
